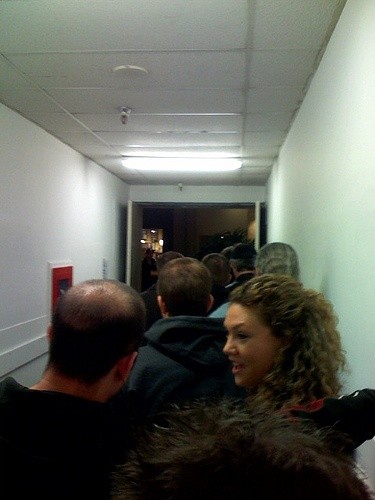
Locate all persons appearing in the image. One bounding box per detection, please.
[138,251,185,317]
[141,248,159,291]
[0,279,171,500]
[109,273,375,500]
[224,243,257,293]
[124,256,243,423]
[201,253,232,315]
